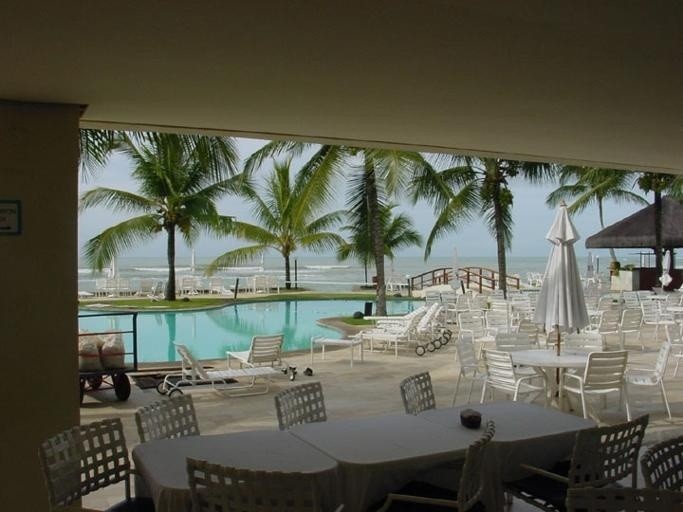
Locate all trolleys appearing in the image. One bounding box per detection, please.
[76,310,139,406]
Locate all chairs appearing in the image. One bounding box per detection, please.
[486,311,517,347]
[157,341,281,403]
[380,302,442,352]
[588,297,613,323]
[623,341,672,422]
[377,417,495,512]
[349,309,435,361]
[496,320,542,352]
[399,371,436,415]
[39,416,159,512]
[480,349,548,410]
[364,306,426,326]
[641,435,683,511]
[505,414,650,512]
[425,289,441,307]
[458,313,483,344]
[639,290,654,312]
[607,308,644,351]
[135,394,201,443]
[638,301,675,340]
[226,335,283,384]
[451,338,510,407]
[584,310,621,351]
[624,292,640,311]
[184,457,320,512]
[561,351,632,426]
[442,290,456,308]
[274,381,327,432]
[566,488,682,512]
[664,326,683,378]
[445,294,467,322]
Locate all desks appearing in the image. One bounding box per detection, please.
[289,413,471,512]
[509,349,588,412]
[310,335,363,367]
[133,430,338,511]
[667,306,683,343]
[418,399,598,505]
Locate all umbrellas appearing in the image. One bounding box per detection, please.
[190,248,197,296]
[586,251,594,283]
[108,254,116,298]
[533,198,590,399]
[450,247,460,290]
[659,250,672,287]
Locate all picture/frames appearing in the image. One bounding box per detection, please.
[0,198,22,237]
